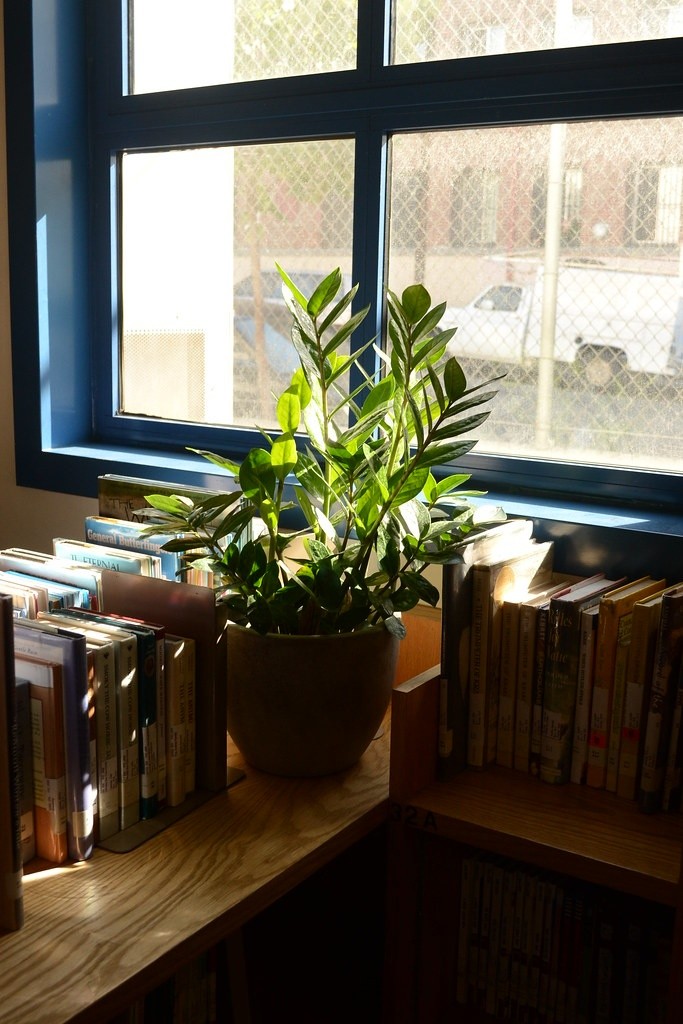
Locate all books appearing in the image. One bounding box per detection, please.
[0,474,269,934]
[436,519,683,810]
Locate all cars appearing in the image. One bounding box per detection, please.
[233,266,350,346]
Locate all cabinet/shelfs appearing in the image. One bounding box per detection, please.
[1,656,683,1024]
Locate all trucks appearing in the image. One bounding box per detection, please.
[420,267,683,397]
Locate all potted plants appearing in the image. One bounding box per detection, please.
[129,263,509,775]
